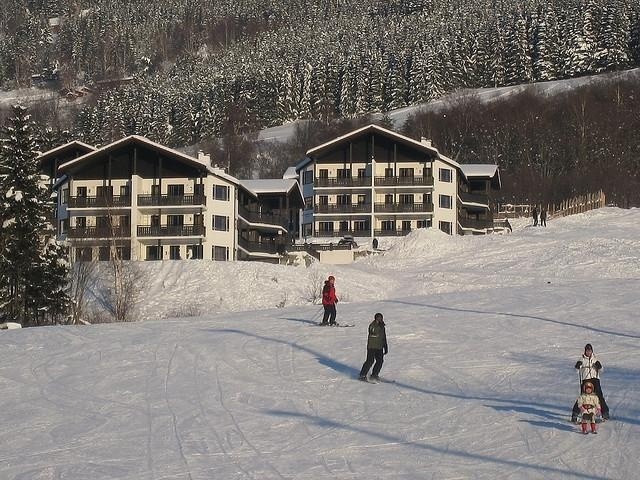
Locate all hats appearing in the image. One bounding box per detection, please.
[584,343,593,351]
[374,312,384,320]
[328,275,335,282]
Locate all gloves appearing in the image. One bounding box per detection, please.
[335,298,338,303]
[327,296,331,300]
[383,345,388,355]
[594,360,602,370]
[574,361,584,370]
[580,406,586,412]
[596,406,602,414]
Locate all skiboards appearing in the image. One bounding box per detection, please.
[358,375,395,384]
[309,321,354,327]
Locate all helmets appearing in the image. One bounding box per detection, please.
[583,381,595,393]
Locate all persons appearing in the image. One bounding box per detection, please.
[319,276,340,325]
[373,239,378,249]
[570,343,610,424]
[358,312,388,381]
[575,381,602,435]
[532,208,538,227]
[540,208,546,227]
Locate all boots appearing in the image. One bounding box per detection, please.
[590,422,598,435]
[581,421,588,435]
